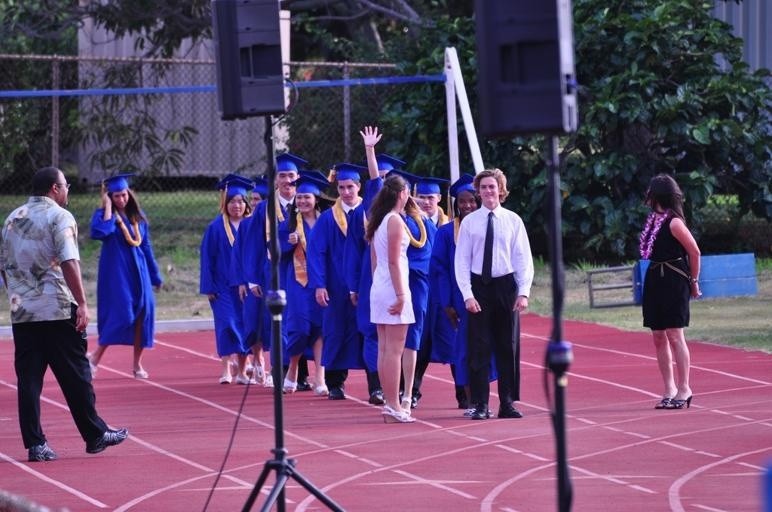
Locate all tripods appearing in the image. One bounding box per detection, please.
[232,114,343,512]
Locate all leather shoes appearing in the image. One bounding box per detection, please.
[283,367,345,401]
[369,390,418,423]
[459,402,523,419]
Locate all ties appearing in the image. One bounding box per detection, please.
[480,212,493,285]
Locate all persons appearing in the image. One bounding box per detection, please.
[200,125,535,424]
[87,176,164,378]
[0,166,129,461]
[637,173,703,409]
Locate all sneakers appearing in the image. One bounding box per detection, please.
[86,427,129,454]
[29,443,58,461]
[86,354,97,377]
[132,369,148,379]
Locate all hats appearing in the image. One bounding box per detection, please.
[376,154,407,170]
[275,153,331,197]
[218,173,269,213]
[380,169,476,224]
[329,162,369,180]
[103,174,133,192]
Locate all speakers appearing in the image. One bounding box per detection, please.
[475,0,578,138]
[212,0,286,119]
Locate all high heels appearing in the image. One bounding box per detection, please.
[655,393,692,408]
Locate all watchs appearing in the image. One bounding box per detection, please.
[691,277,698,284]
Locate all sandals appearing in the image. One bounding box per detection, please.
[218,355,275,388]
